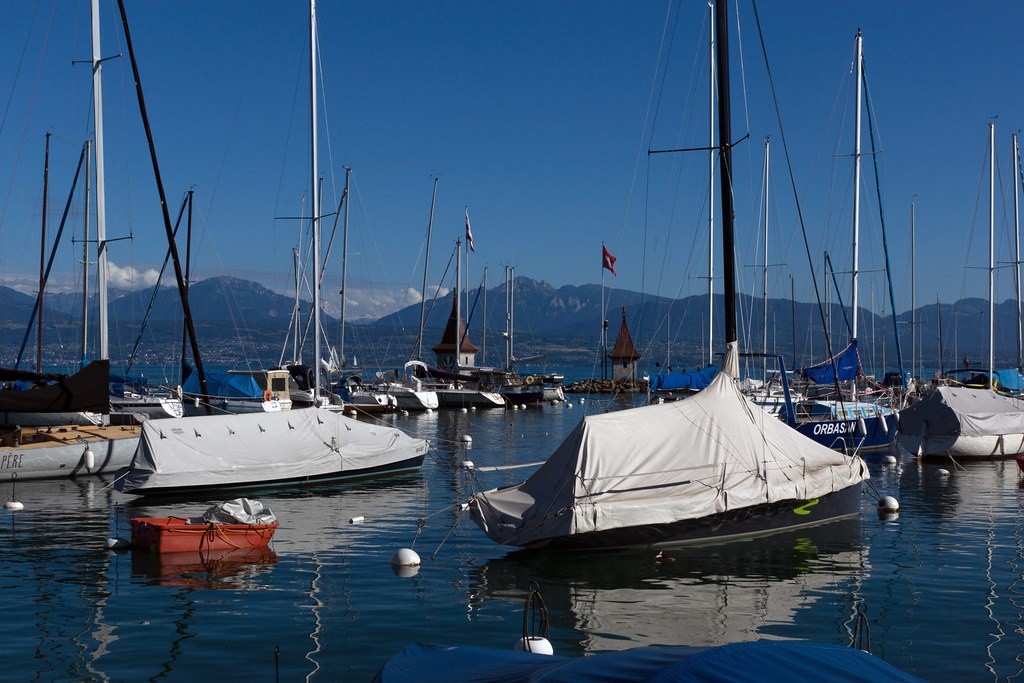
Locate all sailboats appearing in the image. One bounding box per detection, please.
[647,134,898,450]
[466,538,873,654]
[899,120,1024,457]
[900,457,1021,683]
[645,192,965,410]
[461,0,870,573]
[0,0,573,424]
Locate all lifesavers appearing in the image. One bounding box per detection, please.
[526,376,534,385]
[264,390,272,402]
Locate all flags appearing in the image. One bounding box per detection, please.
[602,241,616,277]
[464,206,475,252]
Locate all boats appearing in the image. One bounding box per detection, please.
[114,408,431,497]
[0,425,147,480]
[129,515,279,554]
